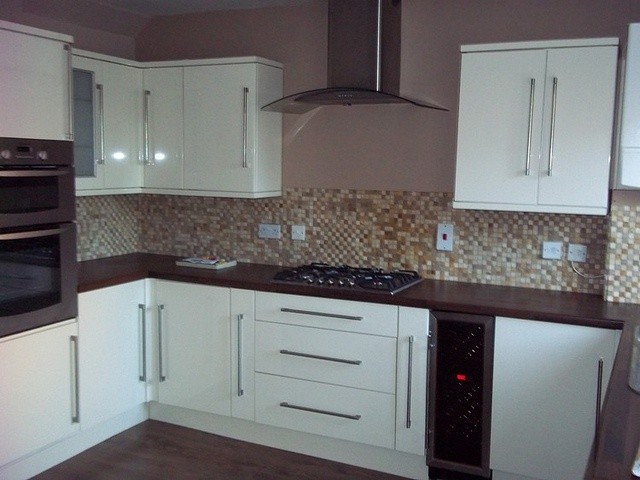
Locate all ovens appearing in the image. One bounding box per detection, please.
[0,137,78,339]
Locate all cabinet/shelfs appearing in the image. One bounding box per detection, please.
[183,56,283,199]
[0,317,80,479]
[150,274,231,436]
[230,285,429,480]
[144,60,184,194]
[611,21,638,191]
[77,278,150,457]
[491,302,621,480]
[452,38,618,215]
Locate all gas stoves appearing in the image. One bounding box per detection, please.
[274,260,423,296]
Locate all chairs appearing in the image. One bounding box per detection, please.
[72,50,144,196]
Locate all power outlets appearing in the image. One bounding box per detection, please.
[258,223,281,240]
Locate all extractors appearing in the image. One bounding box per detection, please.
[260,0,451,114]
